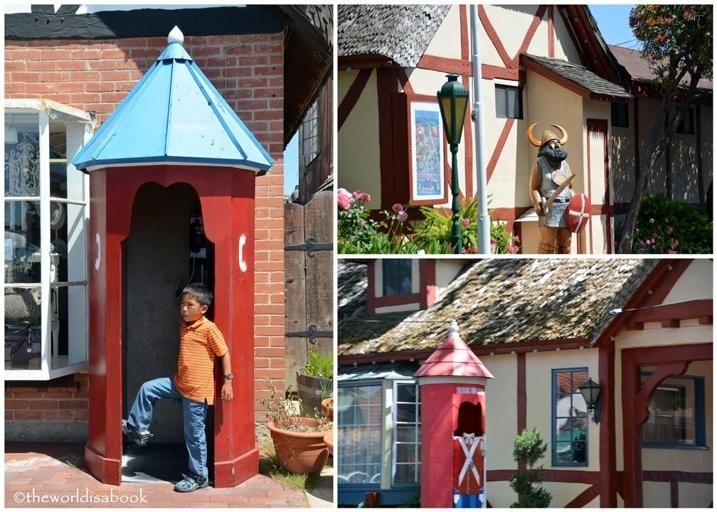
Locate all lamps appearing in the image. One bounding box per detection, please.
[579,377,600,424]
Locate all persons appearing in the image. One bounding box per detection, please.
[122,283,235,492]
[529,129,575,254]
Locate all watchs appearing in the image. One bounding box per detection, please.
[223,373,234,380]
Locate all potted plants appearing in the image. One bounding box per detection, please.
[259,344,333,475]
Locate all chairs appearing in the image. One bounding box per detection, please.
[4,230,60,358]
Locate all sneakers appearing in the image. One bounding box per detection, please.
[122,420,150,446]
[175,472,208,492]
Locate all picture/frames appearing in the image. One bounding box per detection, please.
[392,92,448,205]
